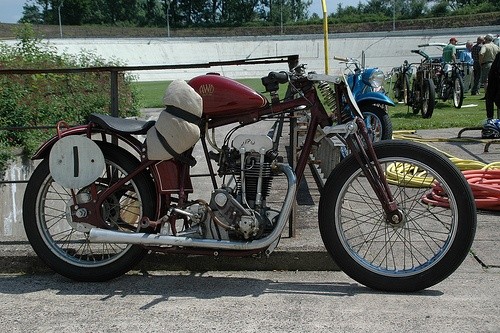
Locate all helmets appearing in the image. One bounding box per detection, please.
[481,118,500,141]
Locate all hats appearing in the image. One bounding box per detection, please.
[484,34,493,41]
[449,37,458,41]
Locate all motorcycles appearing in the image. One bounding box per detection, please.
[22,51,477,294]
[397,54,471,118]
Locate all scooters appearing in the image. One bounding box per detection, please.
[332,55,394,160]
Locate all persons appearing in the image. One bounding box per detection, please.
[460,34,500,95]
[486,52,500,122]
[442,38,458,62]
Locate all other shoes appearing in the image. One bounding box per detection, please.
[470,90,479,95]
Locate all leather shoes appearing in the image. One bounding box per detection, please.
[480,95,487,99]
